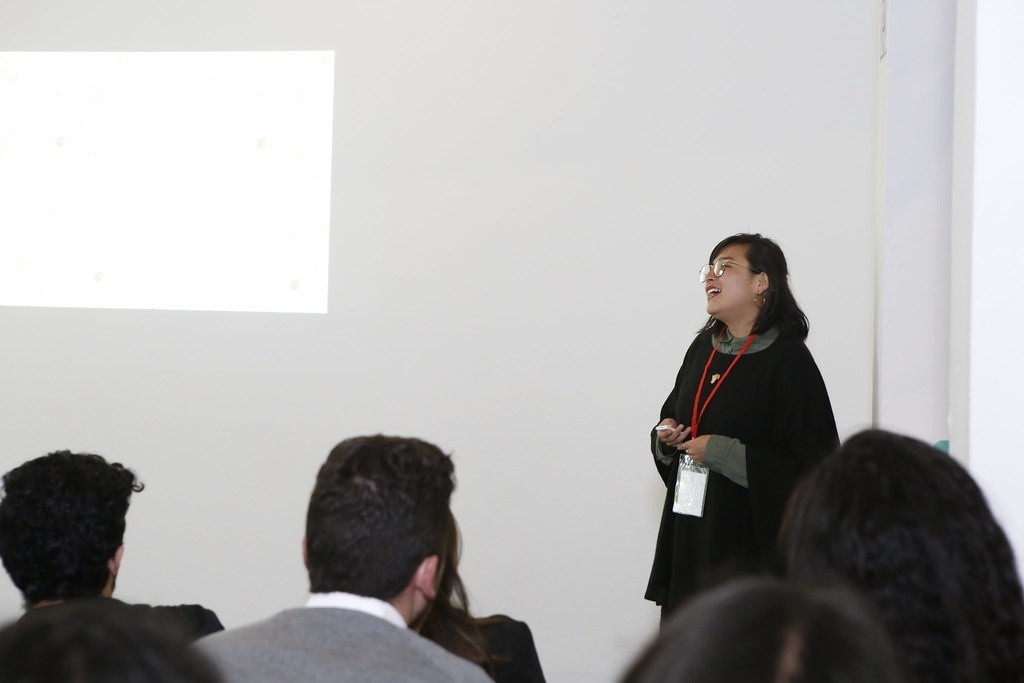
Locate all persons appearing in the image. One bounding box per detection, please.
[0,435,545,683]
[618,429,1024,683]
[644,233,841,631]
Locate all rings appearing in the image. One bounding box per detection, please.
[682,443,688,449]
[685,450,688,453]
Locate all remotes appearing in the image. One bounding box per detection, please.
[655,425,677,432]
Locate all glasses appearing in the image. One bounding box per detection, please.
[699,259,761,283]
